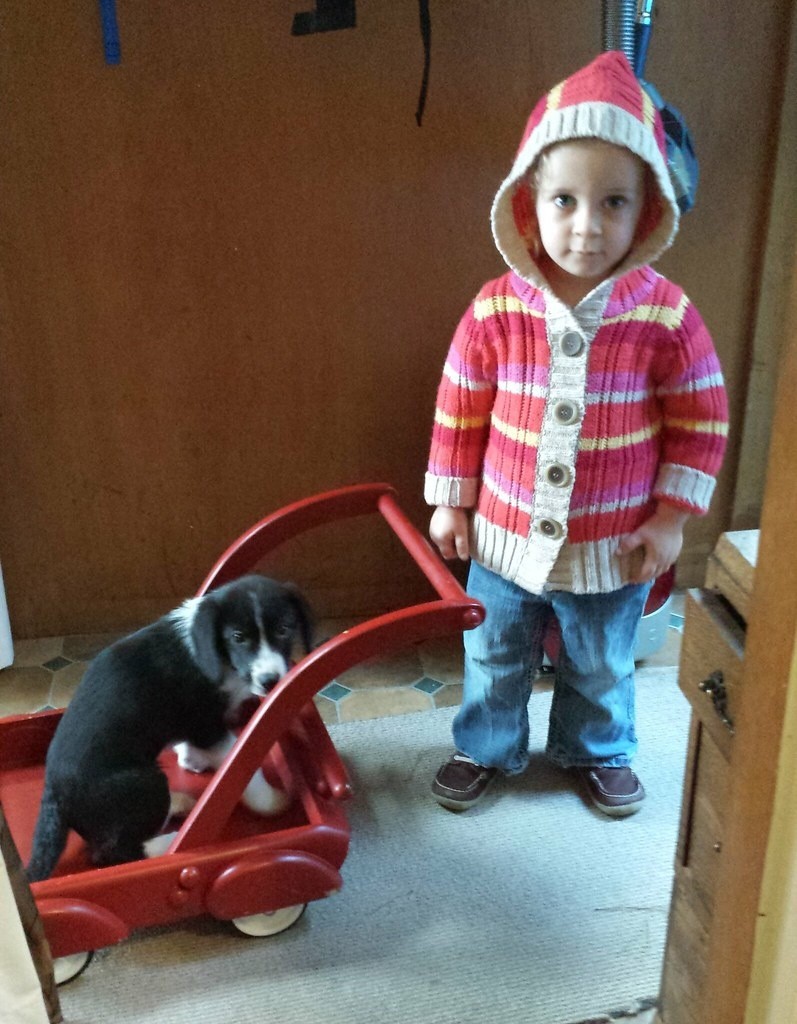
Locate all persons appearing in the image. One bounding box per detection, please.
[424,52,730,822]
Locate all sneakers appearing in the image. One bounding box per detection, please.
[431,749,501,810]
[579,764,647,815]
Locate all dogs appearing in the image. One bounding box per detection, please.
[24,574,318,883]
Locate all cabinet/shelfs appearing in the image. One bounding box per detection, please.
[648,526,760,1024]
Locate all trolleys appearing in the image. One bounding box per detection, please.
[1,476,487,985]
